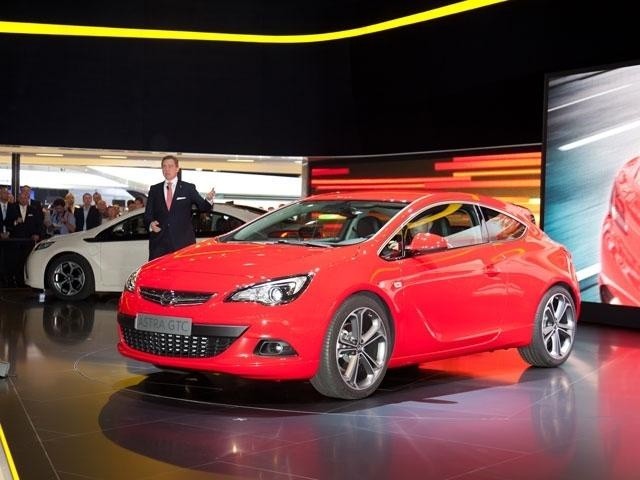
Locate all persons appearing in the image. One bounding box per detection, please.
[0,185,144,288]
[144,156,215,261]
[387,208,446,251]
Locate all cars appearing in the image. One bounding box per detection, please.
[26,288,147,365]
[122,187,268,215]
[24,199,299,305]
[120,190,587,399]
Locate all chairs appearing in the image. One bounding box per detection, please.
[357,216,382,236]
[429,217,452,237]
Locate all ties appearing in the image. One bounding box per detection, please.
[166,182,172,210]
